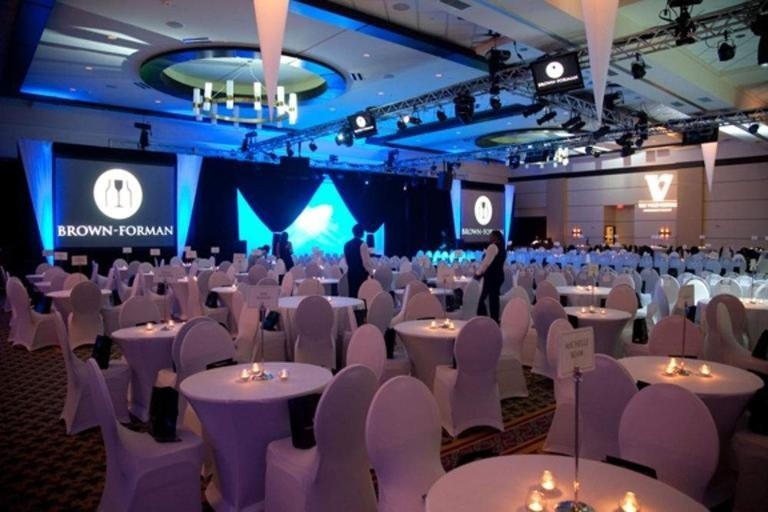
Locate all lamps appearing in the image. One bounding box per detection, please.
[190,62,299,129]
[517,148,572,169]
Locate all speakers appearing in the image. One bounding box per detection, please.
[437,170,452,191]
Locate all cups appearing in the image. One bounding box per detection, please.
[236,361,288,382]
[576,284,608,314]
[142,319,175,332]
[428,317,456,330]
[522,489,547,512]
[537,468,559,494]
[698,362,712,376]
[734,295,764,306]
[451,275,466,281]
[662,357,679,377]
[617,489,643,512]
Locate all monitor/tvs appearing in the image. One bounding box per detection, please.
[530,51,585,96]
[348,111,378,138]
[682,123,719,145]
[524,150,548,164]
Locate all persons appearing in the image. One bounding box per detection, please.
[473,230,505,325]
[344,223,374,321]
[276,232,294,270]
[247,245,269,265]
